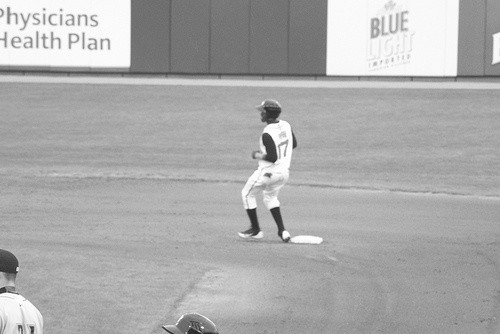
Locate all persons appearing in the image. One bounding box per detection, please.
[238,99,297,243]
[0,249,43,334]
[162,312,220,334]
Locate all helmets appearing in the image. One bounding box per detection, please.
[161,312,217,334]
[255,99,281,117]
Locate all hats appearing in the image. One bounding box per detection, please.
[0,248,19,274]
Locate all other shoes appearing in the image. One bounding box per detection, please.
[278,230,291,242]
[237,228,264,239]
